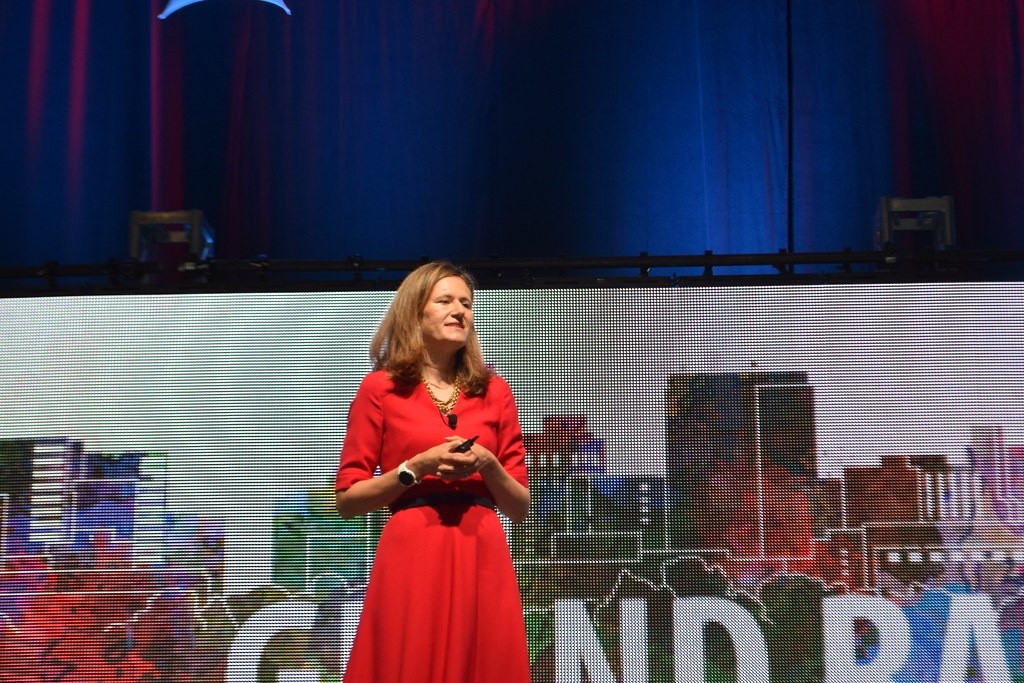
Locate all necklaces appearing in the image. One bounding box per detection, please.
[419,367,461,414]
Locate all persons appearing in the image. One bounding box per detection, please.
[334,261,531,683]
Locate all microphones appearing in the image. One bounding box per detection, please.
[447,412,457,428]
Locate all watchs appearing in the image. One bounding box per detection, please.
[398,460,422,487]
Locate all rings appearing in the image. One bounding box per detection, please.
[463,472,467,478]
